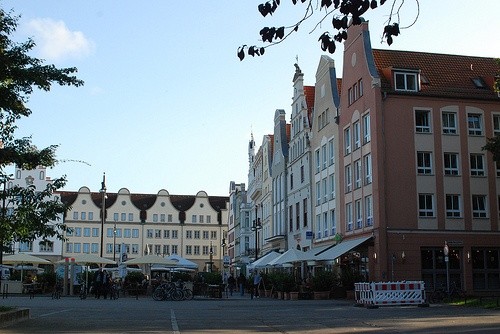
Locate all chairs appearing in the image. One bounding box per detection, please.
[198,282,227,298]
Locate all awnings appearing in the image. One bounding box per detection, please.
[304,237,371,261]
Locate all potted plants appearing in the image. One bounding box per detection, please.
[250,269,343,300]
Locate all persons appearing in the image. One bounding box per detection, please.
[254,270,261,299]
[94,268,110,299]
[228,273,236,296]
[239,272,246,297]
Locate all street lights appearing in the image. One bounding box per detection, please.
[251,205,262,261]
[221,230,227,256]
[98,171,109,266]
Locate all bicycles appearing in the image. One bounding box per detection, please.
[151,279,194,302]
[52,281,63,300]
[76,277,120,300]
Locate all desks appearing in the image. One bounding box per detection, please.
[208,285,219,298]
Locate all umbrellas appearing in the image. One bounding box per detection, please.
[150,255,198,282]
[2,254,52,282]
[251,248,319,281]
[55,253,117,296]
[123,255,176,288]
